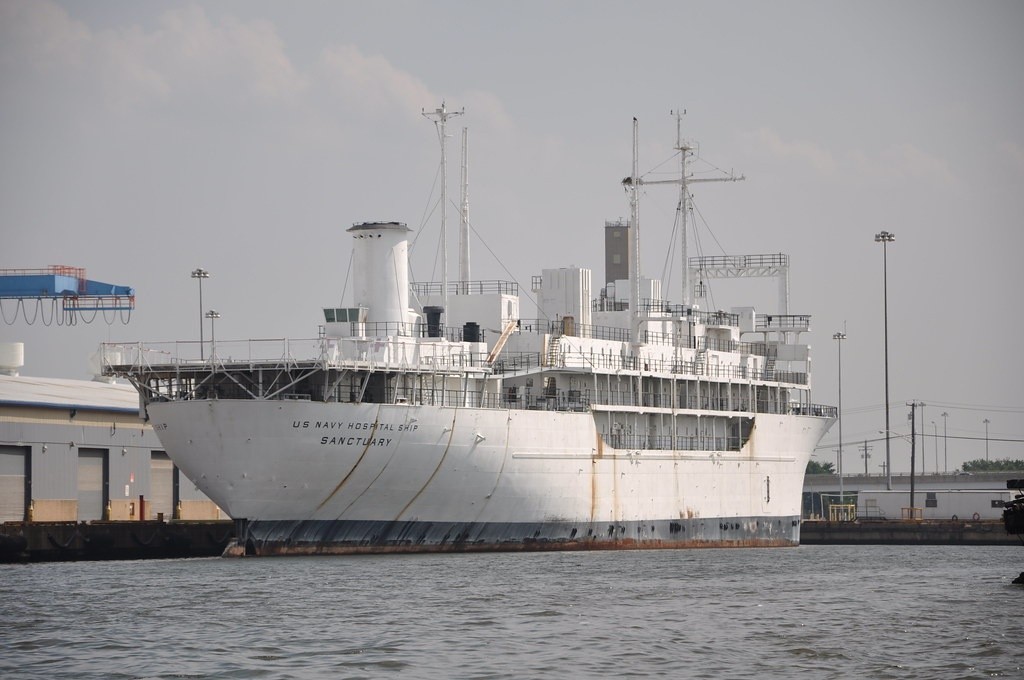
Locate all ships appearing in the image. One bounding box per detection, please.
[101,99,839,554]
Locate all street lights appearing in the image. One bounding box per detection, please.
[191,268,209,361]
[983,419,991,462]
[942,411,949,474]
[878,430,915,521]
[875,229,896,491]
[833,331,847,502]
[931,421,938,474]
[204,309,221,369]
